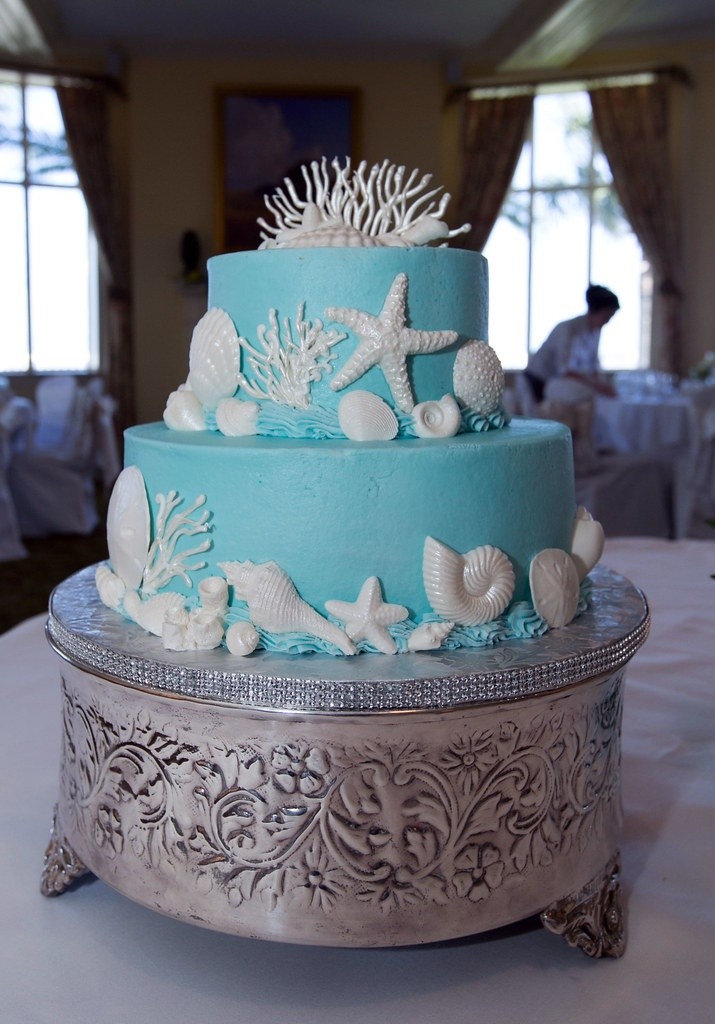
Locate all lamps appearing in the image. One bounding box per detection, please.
[445,60,689,108]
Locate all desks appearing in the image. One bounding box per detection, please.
[1,541,714,1021]
[589,375,702,449]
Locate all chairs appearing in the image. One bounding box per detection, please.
[516,367,715,540]
[0,377,123,560]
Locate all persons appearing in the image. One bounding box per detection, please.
[523,286,620,402]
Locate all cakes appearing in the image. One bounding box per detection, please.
[94,153,604,655]
